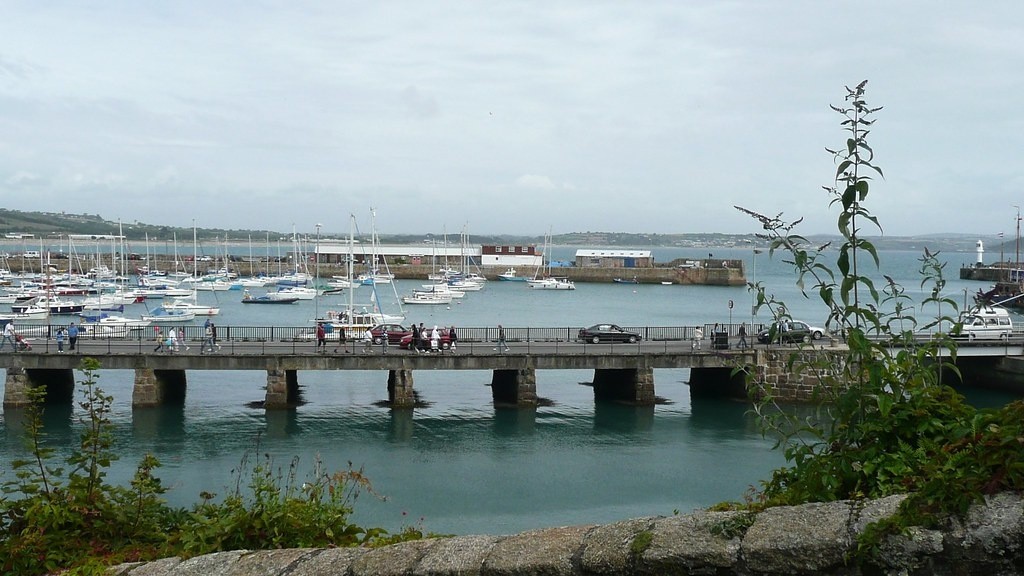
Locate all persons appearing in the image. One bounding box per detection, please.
[710,328,714,348]
[154,326,190,352]
[0,321,29,349]
[783,319,792,331]
[338,312,346,323]
[736,322,749,347]
[362,326,374,353]
[317,323,328,352]
[714,323,719,333]
[200,318,219,354]
[609,326,615,330]
[334,326,350,353]
[69,322,78,350]
[57,330,64,352]
[411,323,457,355]
[493,325,510,351]
[692,326,703,349]
[384,330,389,353]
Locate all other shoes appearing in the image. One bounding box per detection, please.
[61,350,63,352]
[346,350,350,353]
[186,347,190,350]
[58,350,60,352]
[154,349,156,352]
[421,350,425,353]
[334,349,337,352]
[362,349,365,353]
[370,350,374,352]
[493,348,496,351]
[425,351,430,354]
[505,349,510,352]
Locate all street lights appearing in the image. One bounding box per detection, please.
[1013,205,1022,263]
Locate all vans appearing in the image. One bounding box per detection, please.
[947,307,1014,346]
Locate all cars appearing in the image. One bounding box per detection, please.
[779,319,826,340]
[401,329,453,350]
[578,324,642,344]
[756,322,813,345]
[369,323,413,345]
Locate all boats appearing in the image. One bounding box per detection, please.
[612,277,640,285]
[495,268,529,281]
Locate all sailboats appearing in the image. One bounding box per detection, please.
[525,224,576,291]
[401,221,488,304]
[0,207,407,341]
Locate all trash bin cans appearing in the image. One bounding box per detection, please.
[417,257,421,265]
[412,257,417,265]
[715,331,729,350]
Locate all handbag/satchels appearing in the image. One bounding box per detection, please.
[431,340,438,349]
[450,341,456,350]
